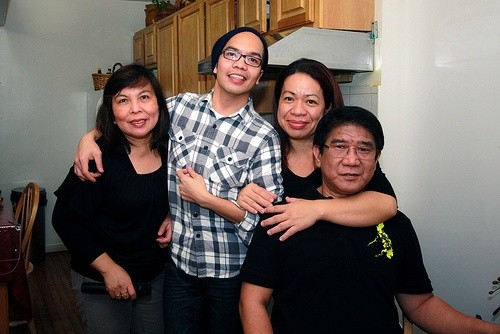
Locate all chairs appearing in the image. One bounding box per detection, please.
[15,182,40,253]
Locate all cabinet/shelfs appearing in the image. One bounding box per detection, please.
[133,0,375,113]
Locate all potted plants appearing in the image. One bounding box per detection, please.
[144,0,196,27]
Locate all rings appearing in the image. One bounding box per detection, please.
[116,293,120,298]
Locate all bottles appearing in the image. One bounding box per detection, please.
[106,69,111,74]
[97,68,102,74]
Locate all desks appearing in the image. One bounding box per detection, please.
[0,196,32,326]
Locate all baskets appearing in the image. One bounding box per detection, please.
[92,62,123,91]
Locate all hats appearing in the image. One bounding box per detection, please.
[211,27,268,80]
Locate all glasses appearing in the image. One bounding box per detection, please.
[220,46,263,67]
[325,142,377,160]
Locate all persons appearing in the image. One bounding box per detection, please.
[238,105,500,334]
[72,28,285,334]
[51,63,174,334]
[236,58,399,241]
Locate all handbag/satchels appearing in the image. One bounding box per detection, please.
[0,224,20,261]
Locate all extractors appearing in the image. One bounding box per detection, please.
[198,25,374,84]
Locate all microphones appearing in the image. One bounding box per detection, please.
[81,281,151,298]
